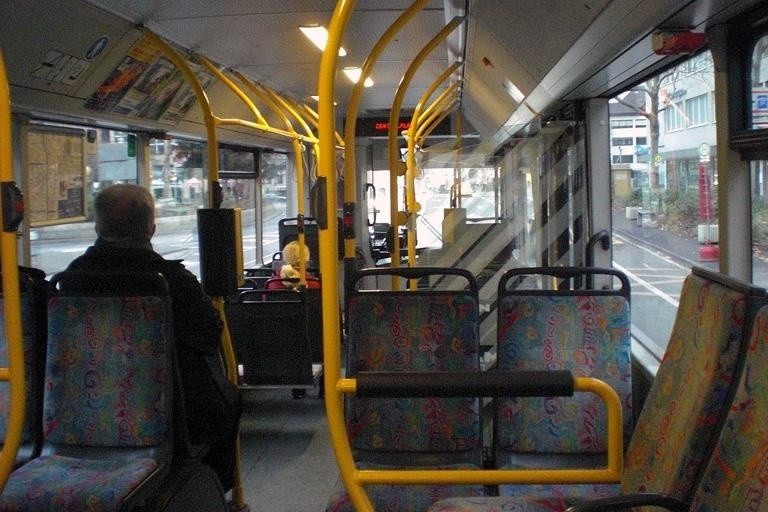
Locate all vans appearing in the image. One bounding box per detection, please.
[453,183,472,197]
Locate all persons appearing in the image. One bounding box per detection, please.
[266,239,320,289]
[59,182,245,491]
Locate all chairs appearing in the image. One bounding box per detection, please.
[224,218,365,397]
[426,269,768,511]
[1,265,246,508]
[490,268,632,495]
[563,303,764,509]
[323,266,488,508]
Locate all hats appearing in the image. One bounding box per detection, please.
[281,239,310,268]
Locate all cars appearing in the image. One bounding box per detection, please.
[264,194,286,203]
